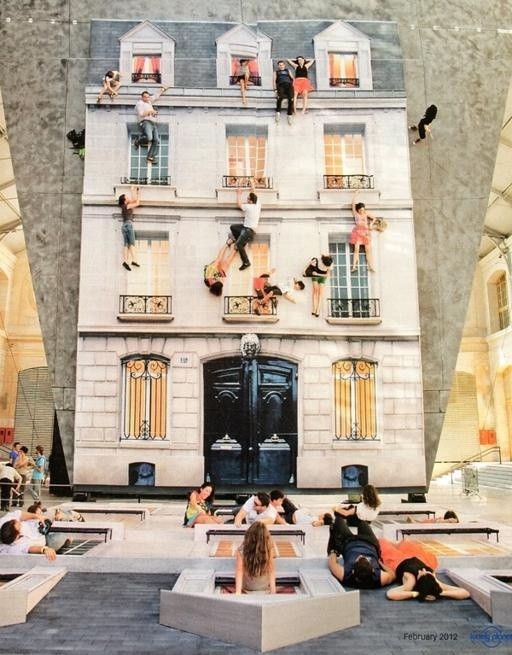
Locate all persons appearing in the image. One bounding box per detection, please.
[97,70,121,101]
[252,194,377,315]
[327,517,397,590]
[408,104,437,145]
[234,521,279,594]
[234,56,314,125]
[0,440,75,561]
[187,482,381,529]
[135,87,167,160]
[377,538,470,602]
[119,190,143,269]
[203,189,263,295]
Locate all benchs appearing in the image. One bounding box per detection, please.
[45,528,113,547]
[204,529,305,550]
[376,510,437,523]
[396,528,499,546]
[74,509,146,522]
[215,512,287,524]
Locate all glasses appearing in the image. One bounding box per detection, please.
[254,500,262,506]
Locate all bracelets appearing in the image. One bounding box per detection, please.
[412,592,416,598]
[330,550,338,555]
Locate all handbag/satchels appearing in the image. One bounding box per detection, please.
[41,472,51,489]
[375,220,388,232]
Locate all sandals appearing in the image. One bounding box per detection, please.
[312,312,316,315]
[315,315,319,318]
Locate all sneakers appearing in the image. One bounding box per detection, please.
[409,126,416,131]
[413,138,420,145]
[134,136,140,150]
[287,115,293,126]
[131,261,140,267]
[122,262,131,271]
[275,112,281,123]
[145,156,158,164]
[239,262,251,271]
